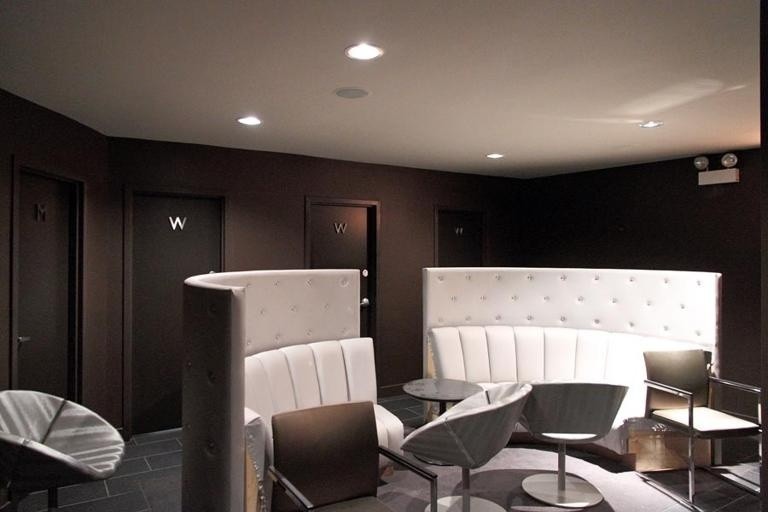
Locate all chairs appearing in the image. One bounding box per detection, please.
[267,401,437,512]
[519,382,630,509]
[642,349,764,508]
[0,391,126,511]
[401,381,533,511]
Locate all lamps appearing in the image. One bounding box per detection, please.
[695,152,740,187]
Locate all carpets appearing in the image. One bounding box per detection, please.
[381,446,694,512]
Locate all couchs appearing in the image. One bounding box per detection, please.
[430,326,713,474]
[243,337,406,512]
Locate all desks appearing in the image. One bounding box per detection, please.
[402,377,484,416]
